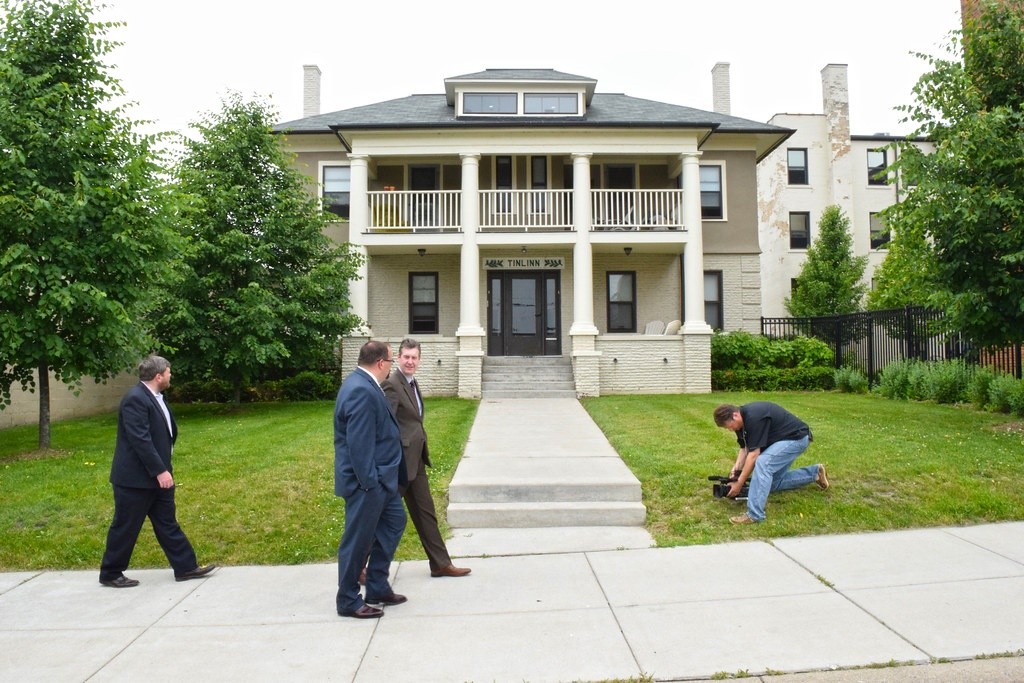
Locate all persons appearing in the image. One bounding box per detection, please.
[333,340,407,618]
[359,338,472,586]
[713,401,829,525]
[99,356,215,587]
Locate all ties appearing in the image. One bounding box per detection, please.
[411,380,416,400]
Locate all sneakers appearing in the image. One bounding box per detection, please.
[728,513,758,526]
[816,464,830,492]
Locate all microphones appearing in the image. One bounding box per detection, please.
[708,476,725,481]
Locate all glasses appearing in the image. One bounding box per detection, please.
[377,359,395,365]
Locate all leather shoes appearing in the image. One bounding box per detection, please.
[431,563,471,577]
[98,574,139,588]
[365,588,407,605]
[175,564,216,581]
[358,565,367,585]
[337,601,384,618]
